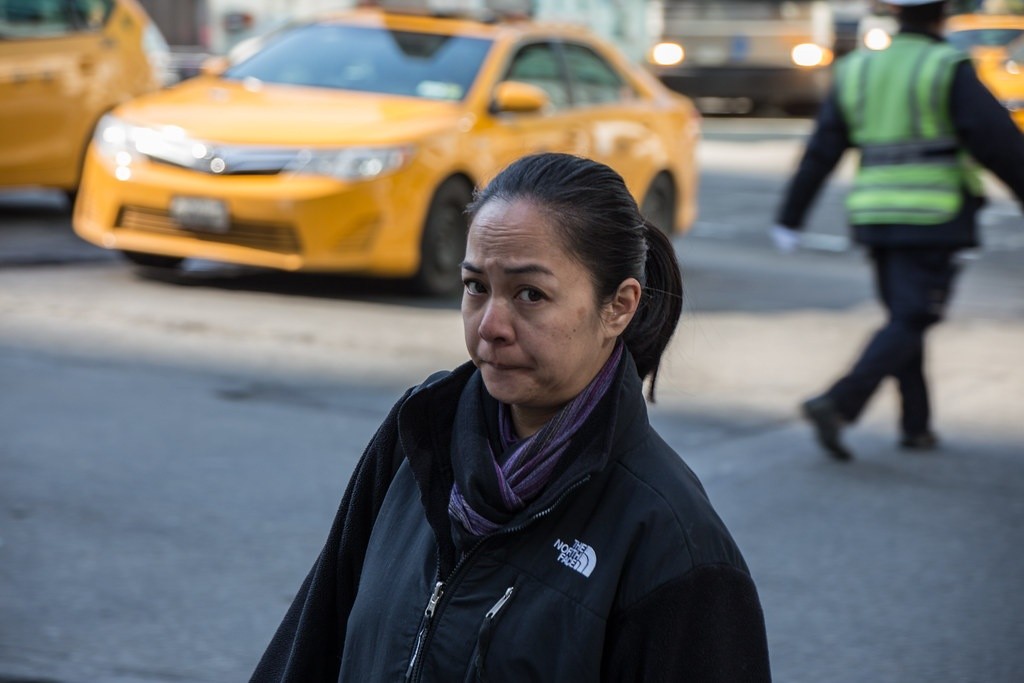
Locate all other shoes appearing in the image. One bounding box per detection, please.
[895,429,939,453]
[801,395,856,463]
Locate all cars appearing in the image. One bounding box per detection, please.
[940,13,1024,131]
[0,0,703,301]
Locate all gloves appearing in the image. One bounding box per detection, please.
[768,224,805,255]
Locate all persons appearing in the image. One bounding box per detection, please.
[248,153,773,683]
[770,0,1024,461]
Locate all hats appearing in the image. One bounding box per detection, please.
[883,0,947,13]
